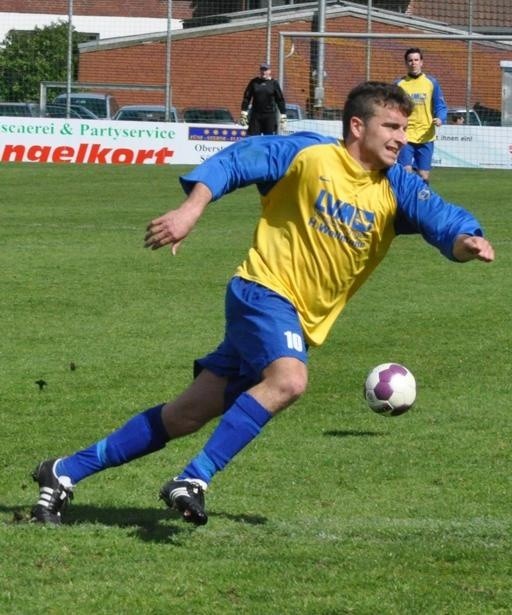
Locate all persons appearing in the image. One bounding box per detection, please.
[394,48,447,187]
[451,112,465,125]
[241,64,287,135]
[30,80,495,524]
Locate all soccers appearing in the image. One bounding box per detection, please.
[363,363,416,417]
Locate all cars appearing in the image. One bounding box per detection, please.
[244,103,303,121]
[446,107,482,126]
[178,107,238,122]
[54,93,119,119]
[111,104,178,122]
[0,102,98,119]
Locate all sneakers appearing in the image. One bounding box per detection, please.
[159,476,207,525]
[31,457,76,525]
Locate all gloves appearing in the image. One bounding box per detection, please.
[281,118,286,129]
[240,114,248,125]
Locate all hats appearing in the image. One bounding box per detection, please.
[260,63,271,68]
